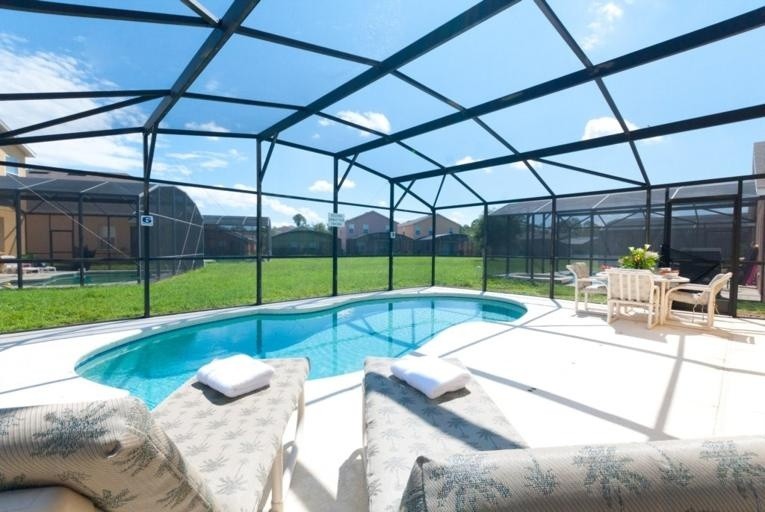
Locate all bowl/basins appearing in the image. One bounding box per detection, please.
[663,272,679,277]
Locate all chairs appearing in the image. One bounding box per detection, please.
[1,357,309,511]
[362,355,765,509]
[567,263,732,331]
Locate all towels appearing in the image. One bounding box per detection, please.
[388,351,470,400]
[198,353,274,399]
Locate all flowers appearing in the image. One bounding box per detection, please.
[618,244,661,270]
